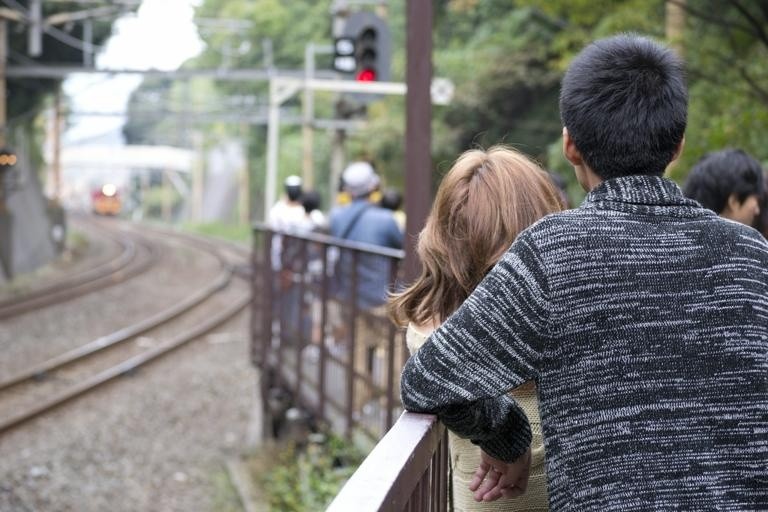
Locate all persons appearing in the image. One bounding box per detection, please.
[400,31,768,512]
[684,148,764,226]
[383,142,568,512]
[267,161,406,412]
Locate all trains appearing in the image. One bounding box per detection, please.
[92,185,121,216]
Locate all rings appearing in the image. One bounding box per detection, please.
[492,467,501,473]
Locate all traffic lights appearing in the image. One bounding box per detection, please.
[0,150,17,171]
[333,28,378,85]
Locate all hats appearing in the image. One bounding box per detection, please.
[342,162,379,195]
